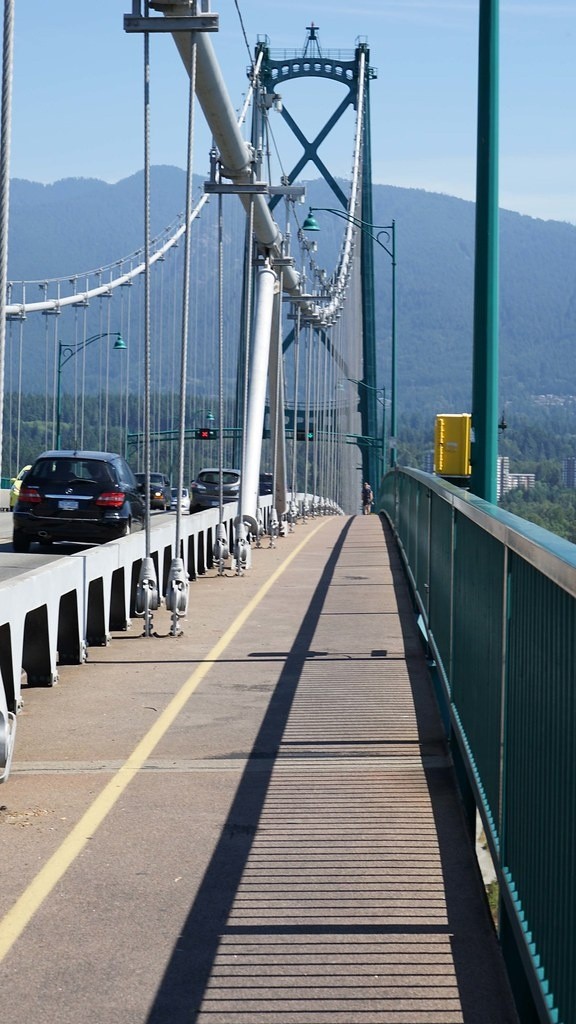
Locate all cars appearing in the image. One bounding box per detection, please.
[188,467,241,515]
[133,472,173,512]
[258,473,274,496]
[170,487,191,511]
[10,465,33,511]
[12,449,148,552]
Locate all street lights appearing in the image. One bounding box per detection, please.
[301,205,398,467]
[333,376,387,478]
[57,331,128,450]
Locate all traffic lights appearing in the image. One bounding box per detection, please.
[196,429,216,440]
[297,431,313,441]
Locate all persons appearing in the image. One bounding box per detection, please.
[361,482,374,514]
